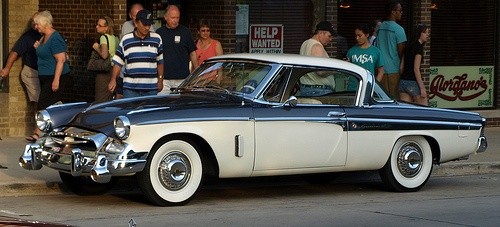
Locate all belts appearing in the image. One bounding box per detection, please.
[300,84,332,90]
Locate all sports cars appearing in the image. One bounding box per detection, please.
[18,51,488,208]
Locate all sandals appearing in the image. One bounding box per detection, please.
[24,133,40,143]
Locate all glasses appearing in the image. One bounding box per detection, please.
[200,29,210,32]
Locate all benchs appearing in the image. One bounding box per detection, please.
[310,96,357,105]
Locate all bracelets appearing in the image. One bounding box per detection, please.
[158,75,164,79]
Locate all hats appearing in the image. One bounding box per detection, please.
[135,9,155,26]
[316,20,337,37]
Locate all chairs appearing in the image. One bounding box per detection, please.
[296,98,323,104]
[241,79,257,94]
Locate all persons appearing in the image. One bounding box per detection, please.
[398,24,429,107]
[91,16,120,102]
[298,21,342,96]
[24,10,73,140]
[115,4,156,99]
[108,9,165,98]
[368,19,382,47]
[343,26,385,91]
[190,22,224,87]
[0,20,64,105]
[375,2,407,101]
[155,4,198,96]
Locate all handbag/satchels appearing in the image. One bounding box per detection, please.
[86,34,111,73]
